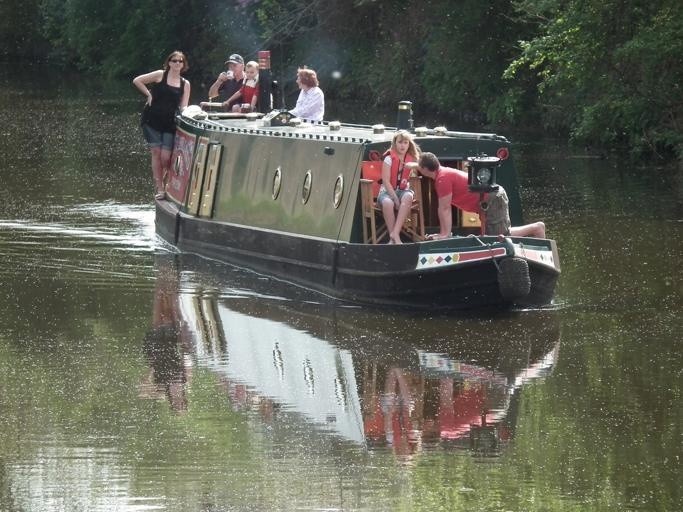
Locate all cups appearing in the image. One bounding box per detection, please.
[226,69,234,80]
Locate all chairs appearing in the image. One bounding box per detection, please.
[359,160,426,244]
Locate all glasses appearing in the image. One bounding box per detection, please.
[172,60,183,63]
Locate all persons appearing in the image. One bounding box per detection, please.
[133,50,191,200]
[225,60,260,112]
[417,150,546,240]
[144,280,196,414]
[208,53,246,113]
[288,64,325,121]
[362,366,513,469]
[376,127,422,244]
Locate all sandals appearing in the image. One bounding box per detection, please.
[155,191,165,200]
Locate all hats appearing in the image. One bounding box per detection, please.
[225,54,244,63]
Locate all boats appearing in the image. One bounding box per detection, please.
[155,51,563,305]
